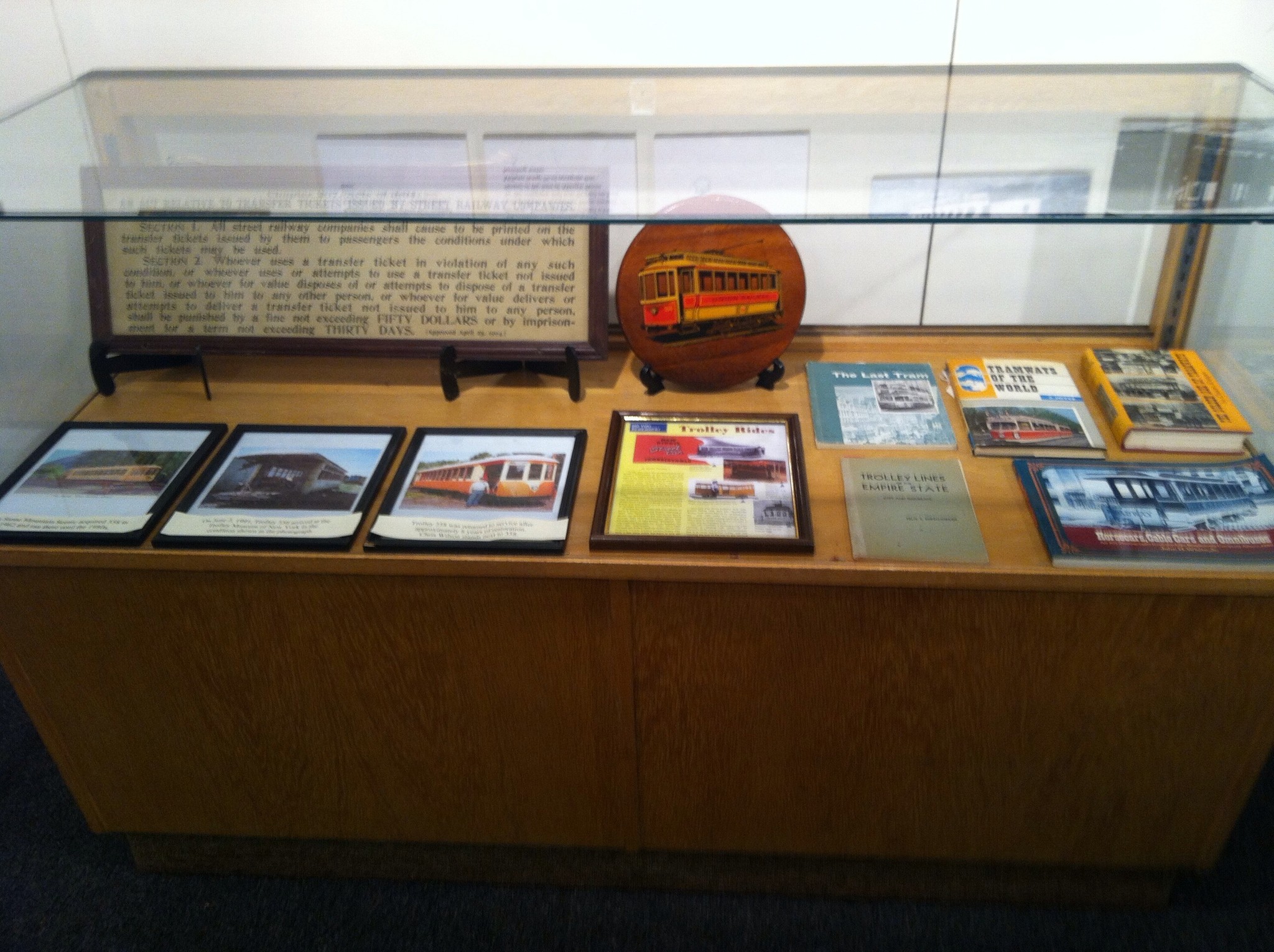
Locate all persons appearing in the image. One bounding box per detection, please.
[464,476,492,508]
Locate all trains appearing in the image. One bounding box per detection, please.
[1102,476,1255,536]
[64,464,161,487]
[695,484,755,500]
[698,442,764,460]
[411,452,560,504]
[635,249,784,348]
[215,452,349,506]
[985,414,1073,446]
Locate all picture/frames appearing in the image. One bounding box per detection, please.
[362,425,585,558]
[590,406,817,552]
[153,424,408,557]
[79,163,609,368]
[0,418,226,552]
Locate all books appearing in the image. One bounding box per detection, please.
[801,345,1274,570]
